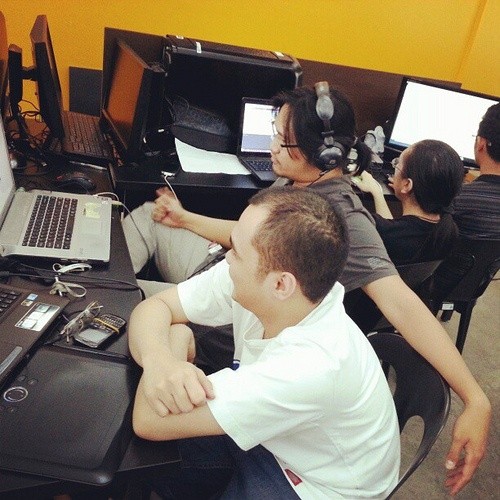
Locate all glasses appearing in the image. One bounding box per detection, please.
[271,121,297,147]
[472,130,491,146]
[64,301,103,343]
[390,158,414,188]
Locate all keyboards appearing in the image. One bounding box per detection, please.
[61,111,113,164]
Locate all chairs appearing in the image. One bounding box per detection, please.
[344,231,499,500]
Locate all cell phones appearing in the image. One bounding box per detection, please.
[162,166,181,176]
[74,312,127,350]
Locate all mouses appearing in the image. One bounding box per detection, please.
[54,171,96,192]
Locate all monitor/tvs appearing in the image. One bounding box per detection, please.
[384,77,500,168]
[8,13,65,149]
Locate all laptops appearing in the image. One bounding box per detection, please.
[0,284,70,386]
[238,96,278,183]
[0,115,112,264]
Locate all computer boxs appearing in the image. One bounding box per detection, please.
[162,45,303,133]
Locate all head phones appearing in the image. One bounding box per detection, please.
[314,80,346,170]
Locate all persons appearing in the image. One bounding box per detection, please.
[125,184,399,500]
[344,139,465,330]
[122,85,492,500]
[448,101,500,310]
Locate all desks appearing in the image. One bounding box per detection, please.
[0,117,183,500]
[109,165,481,222]
